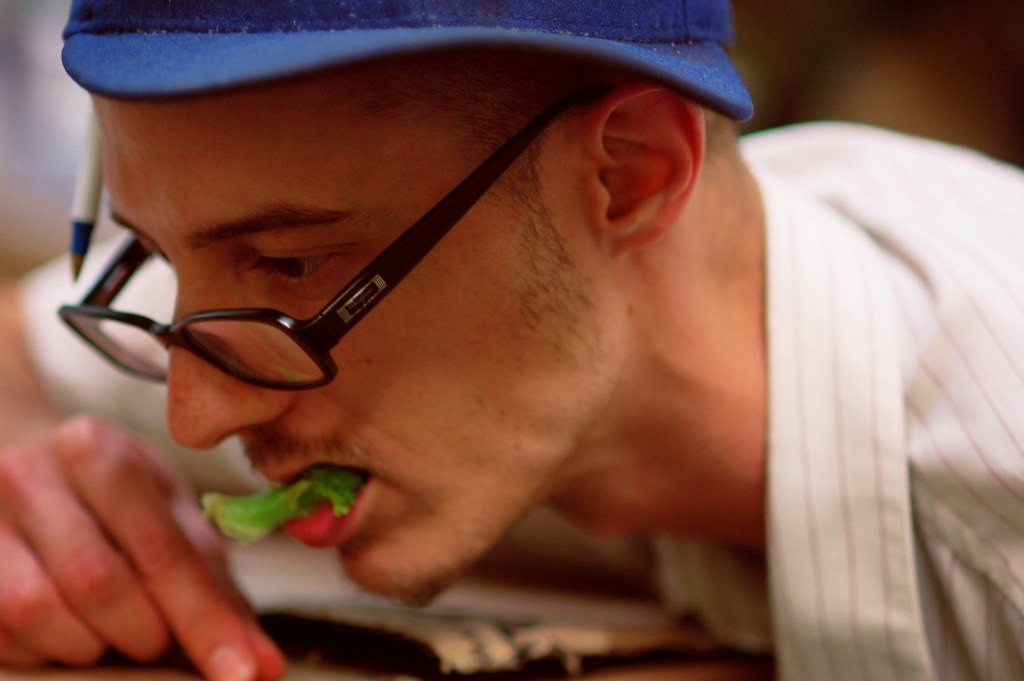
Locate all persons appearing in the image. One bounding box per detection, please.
[0,0,1024,681]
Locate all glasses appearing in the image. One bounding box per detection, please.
[58,84,610,390]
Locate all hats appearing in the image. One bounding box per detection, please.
[61,0,754,122]
